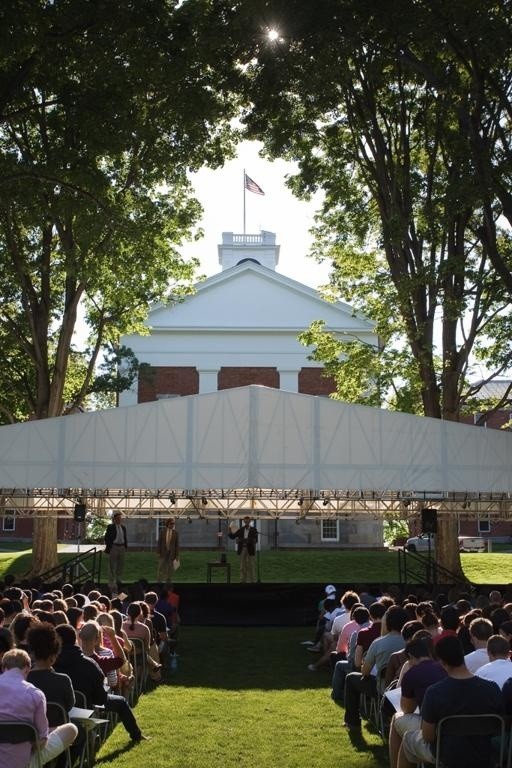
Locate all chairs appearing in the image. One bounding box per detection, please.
[347,630,512,768]
[1,626,160,768]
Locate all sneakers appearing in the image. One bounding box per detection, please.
[307,646,320,652]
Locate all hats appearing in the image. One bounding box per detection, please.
[325,585,336,594]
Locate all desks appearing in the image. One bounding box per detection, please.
[207,560,231,583]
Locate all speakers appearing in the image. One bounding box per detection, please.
[73,504,85,521]
[421,509,437,534]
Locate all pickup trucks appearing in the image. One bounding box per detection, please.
[405,532,487,554]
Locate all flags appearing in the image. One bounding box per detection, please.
[246,175,265,196]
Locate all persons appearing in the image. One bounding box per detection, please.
[105,512,127,589]
[301,585,512,768]
[228,516,261,583]
[0,575,179,767]
[157,519,179,583]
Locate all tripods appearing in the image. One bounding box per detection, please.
[410,540,445,583]
[65,526,96,580]
